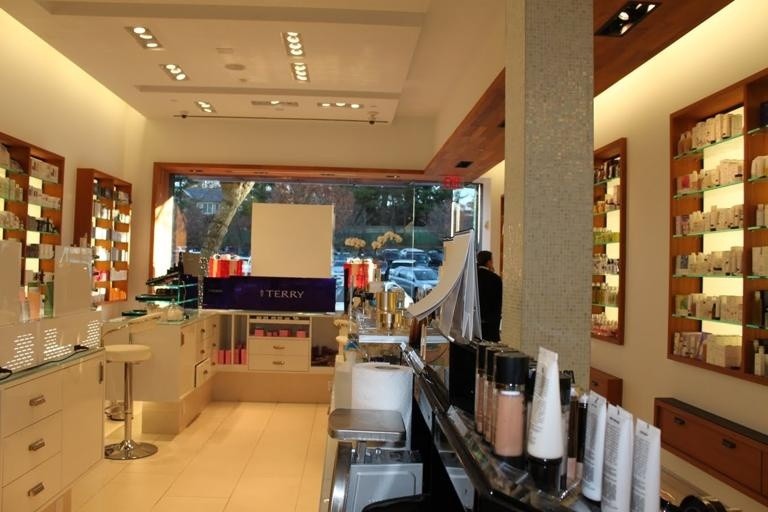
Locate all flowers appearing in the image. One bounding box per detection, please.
[344,231,403,258]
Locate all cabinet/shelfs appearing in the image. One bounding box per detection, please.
[0,132,132,306]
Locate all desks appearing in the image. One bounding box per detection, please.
[358,281,450,345]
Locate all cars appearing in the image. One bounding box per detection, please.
[334,248,444,311]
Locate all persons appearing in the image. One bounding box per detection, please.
[476,251,501,342]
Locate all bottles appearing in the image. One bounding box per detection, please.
[473,338,528,461]
[27,280,53,320]
